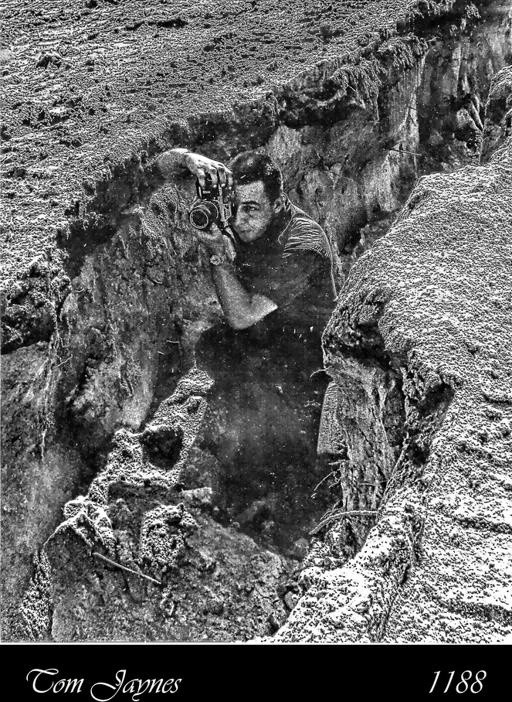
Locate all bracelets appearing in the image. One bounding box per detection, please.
[181,151,191,168]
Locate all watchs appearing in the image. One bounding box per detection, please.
[210,254,230,266]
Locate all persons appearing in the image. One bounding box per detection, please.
[154,146,339,392]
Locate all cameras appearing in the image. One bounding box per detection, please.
[190,181,236,245]
